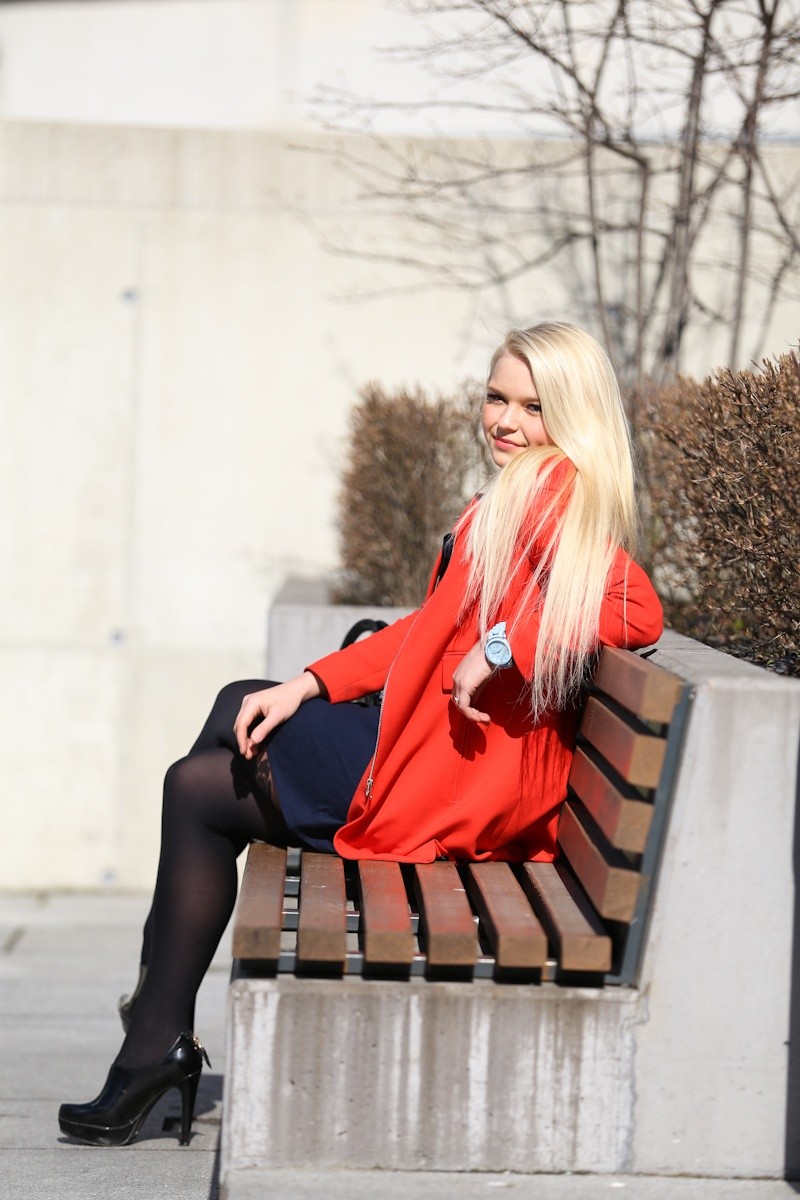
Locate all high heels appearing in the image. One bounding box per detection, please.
[57,1032,212,1146]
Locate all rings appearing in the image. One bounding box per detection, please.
[454,696,460,706]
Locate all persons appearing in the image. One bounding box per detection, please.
[57,321,664,1147]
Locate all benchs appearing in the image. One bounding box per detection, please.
[209,605,800,1200]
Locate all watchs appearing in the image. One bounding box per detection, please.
[484,621,513,668]
